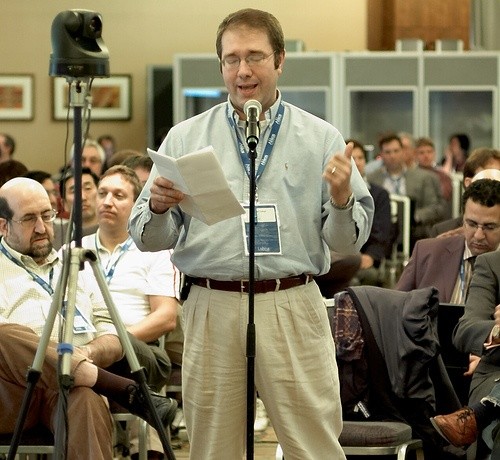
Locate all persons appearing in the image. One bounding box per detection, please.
[253,132,500,460]
[127,8,374,460]
[0,133,188,460]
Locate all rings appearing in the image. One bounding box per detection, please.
[331,167,336,174]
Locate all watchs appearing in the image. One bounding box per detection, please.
[78,345,92,357]
[493,325,500,344]
[330,191,354,209]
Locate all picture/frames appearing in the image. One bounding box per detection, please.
[51,74,132,121]
[0,72,36,121]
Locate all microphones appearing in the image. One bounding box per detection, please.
[243,99,262,149]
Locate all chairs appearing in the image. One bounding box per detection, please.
[276,298,425,460]
[381,194,411,288]
[0,335,167,460]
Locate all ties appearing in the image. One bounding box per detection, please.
[464,255,477,304]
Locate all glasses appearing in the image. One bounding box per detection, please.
[5,209,58,228]
[218,50,276,70]
[463,216,500,233]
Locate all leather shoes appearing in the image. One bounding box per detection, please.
[430,406,478,451]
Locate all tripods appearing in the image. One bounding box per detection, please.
[5,85,177,460]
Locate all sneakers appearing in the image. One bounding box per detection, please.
[125,384,178,430]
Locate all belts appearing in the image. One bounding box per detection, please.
[191,273,313,293]
